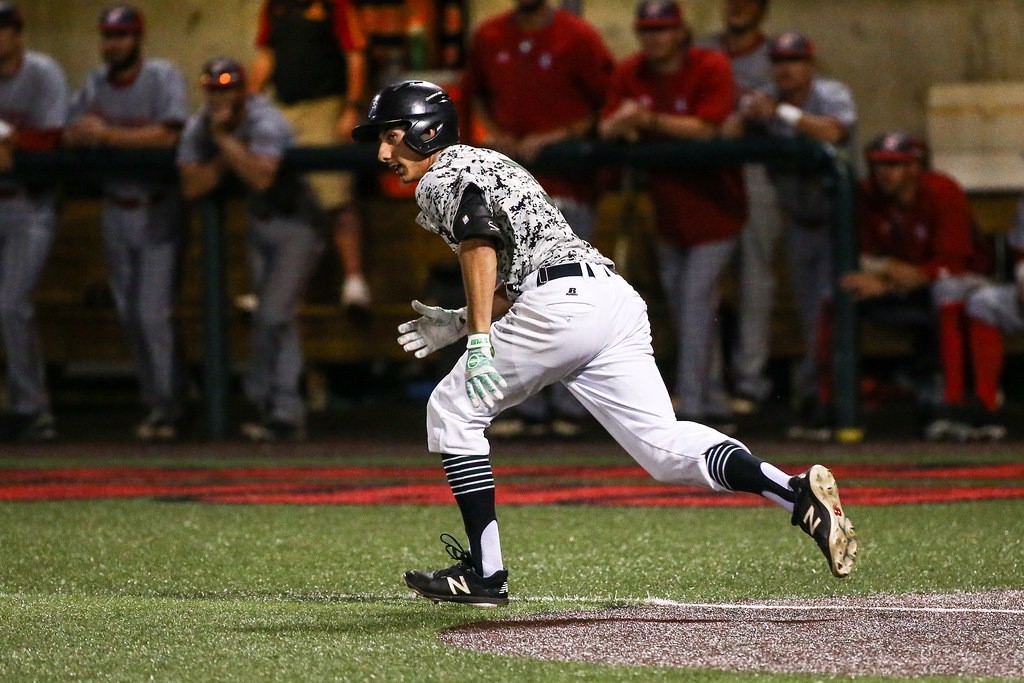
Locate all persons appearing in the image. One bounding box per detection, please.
[235,0,371,315]
[63,4,190,441]
[602,0,1024,444]
[174,56,322,445]
[352,79,859,608]
[470,0,616,439]
[0,0,72,440]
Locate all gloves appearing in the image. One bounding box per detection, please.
[397,299,468,359]
[465,332,507,408]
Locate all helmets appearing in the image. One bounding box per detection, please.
[352,79,458,155]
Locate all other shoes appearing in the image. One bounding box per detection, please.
[140,410,172,442]
[243,404,307,445]
[27,413,58,441]
[341,274,370,309]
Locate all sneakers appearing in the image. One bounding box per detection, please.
[405,534,509,607]
[789,464,858,577]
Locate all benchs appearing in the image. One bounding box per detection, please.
[36,198,1024,377]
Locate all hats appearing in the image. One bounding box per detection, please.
[770,29,814,62]
[200,58,244,90]
[634,0,683,30]
[865,129,930,164]
[99,5,143,36]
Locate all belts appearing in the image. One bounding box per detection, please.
[537,263,609,286]
